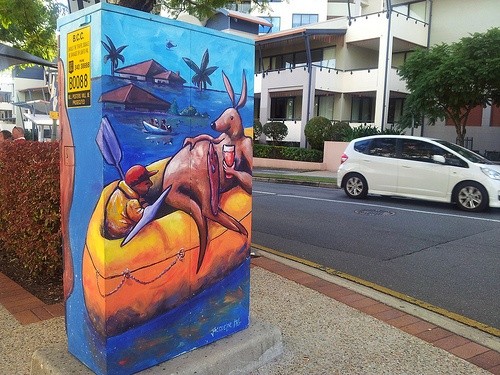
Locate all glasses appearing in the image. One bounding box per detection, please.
[14,126,20,132]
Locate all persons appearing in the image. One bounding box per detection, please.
[12,127,25,141]
[0,130,12,142]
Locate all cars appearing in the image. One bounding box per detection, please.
[337,135,500,212]
